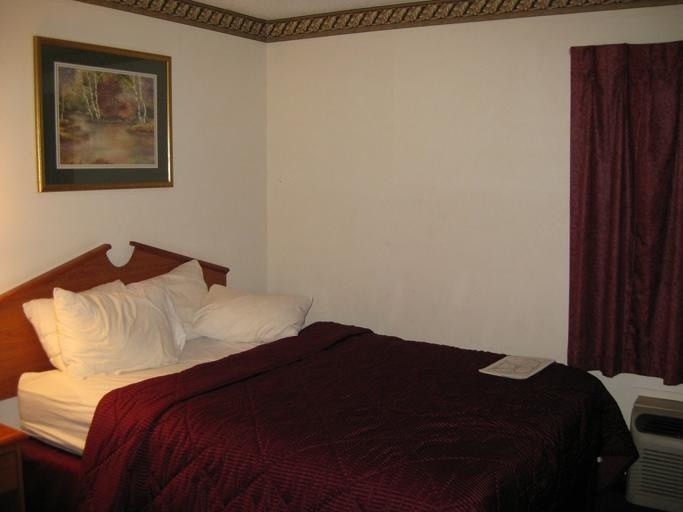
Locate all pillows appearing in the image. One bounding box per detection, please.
[22,260,312,391]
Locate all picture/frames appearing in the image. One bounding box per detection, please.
[33,35,173,192]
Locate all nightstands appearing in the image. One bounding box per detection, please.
[1,423,29,512]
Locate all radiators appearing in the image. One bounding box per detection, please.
[627,394,682,511]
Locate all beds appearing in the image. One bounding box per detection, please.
[0,239,640,510]
